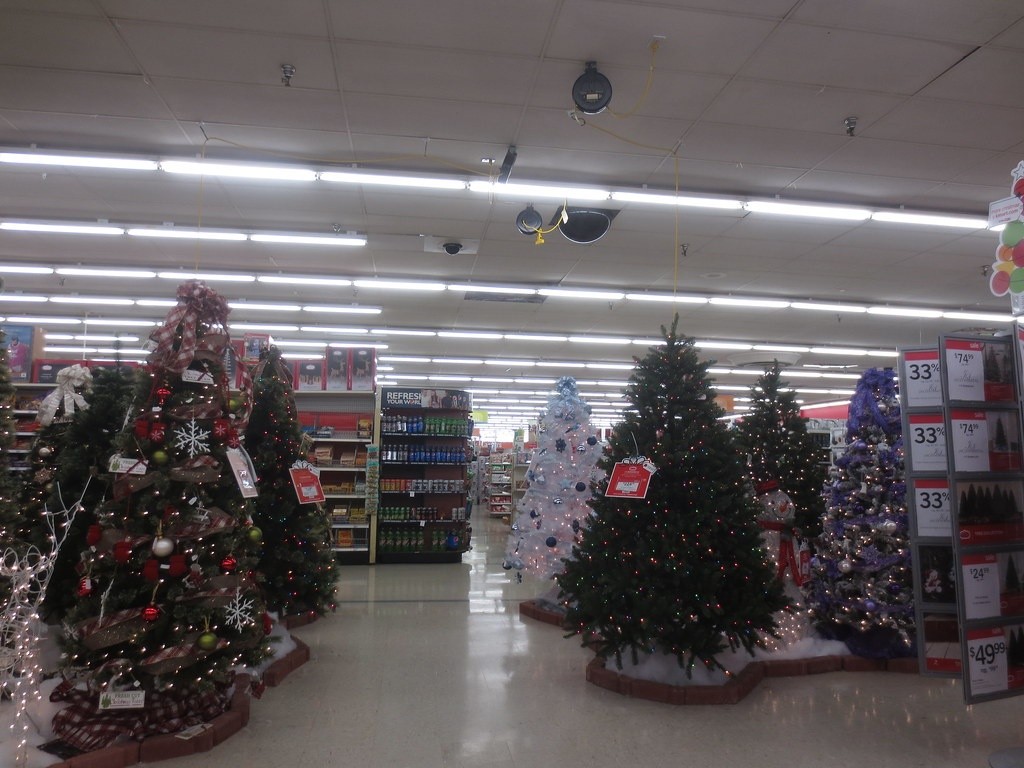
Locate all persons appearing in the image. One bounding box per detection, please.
[7,336,31,372]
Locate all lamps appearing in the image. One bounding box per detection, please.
[0,143,1024,430]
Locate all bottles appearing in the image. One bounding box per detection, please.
[465,496,473,518]
[381,444,472,463]
[380,479,464,491]
[431,526,472,552]
[451,506,465,520]
[467,469,473,491]
[377,506,438,520]
[381,414,474,436]
[377,526,425,553]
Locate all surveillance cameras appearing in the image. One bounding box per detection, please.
[443,243,462,255]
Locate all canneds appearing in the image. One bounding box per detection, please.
[379,507,438,521]
[378,479,465,491]
[452,507,466,520]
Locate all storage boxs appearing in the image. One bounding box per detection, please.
[468,454,489,505]
[342,482,353,494]
[492,473,505,483]
[285,360,296,390]
[495,497,501,503]
[500,497,506,503]
[505,497,511,503]
[19,397,42,411]
[15,421,40,432]
[18,440,32,450]
[355,484,366,495]
[517,499,522,505]
[226,361,251,391]
[340,451,354,467]
[10,441,18,449]
[512,511,520,524]
[231,339,245,360]
[513,499,517,505]
[0,324,47,384]
[86,360,138,370]
[495,506,502,512]
[10,459,31,467]
[333,508,367,524]
[349,347,376,392]
[324,346,349,391]
[243,332,275,363]
[338,530,352,548]
[490,453,502,463]
[356,418,371,438]
[308,446,335,467]
[301,424,336,438]
[33,358,86,384]
[500,507,505,512]
[490,506,495,512]
[356,451,367,468]
[492,465,504,471]
[322,485,338,495]
[296,359,325,390]
[490,496,495,503]
[518,452,531,464]
[516,480,530,489]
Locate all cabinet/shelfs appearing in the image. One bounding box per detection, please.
[11,383,59,471]
[226,387,383,566]
[511,453,531,528]
[377,387,473,565]
[488,452,512,518]
